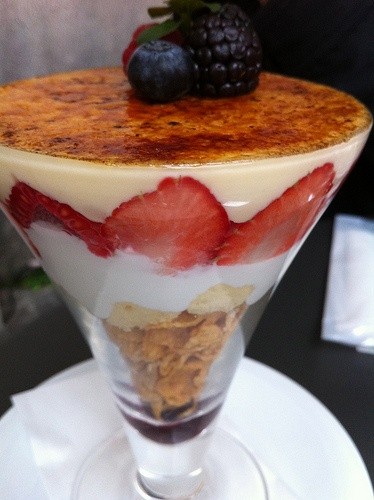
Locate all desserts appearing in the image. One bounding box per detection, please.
[0,61,373,418]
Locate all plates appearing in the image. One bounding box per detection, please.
[0,357,373,499]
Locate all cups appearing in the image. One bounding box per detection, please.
[0,73,372,499]
